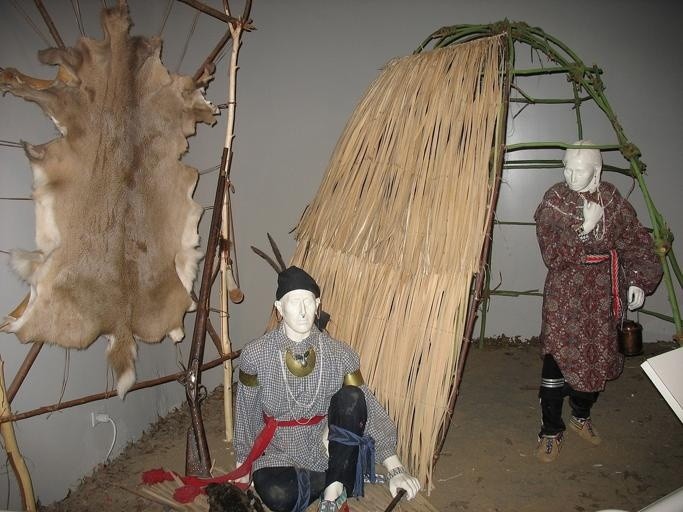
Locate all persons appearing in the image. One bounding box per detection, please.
[232,264,422,511]
[535,138,663,464]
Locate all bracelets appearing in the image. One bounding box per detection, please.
[384,466,407,479]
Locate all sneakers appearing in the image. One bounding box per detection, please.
[316,484,350,512]
[536,430,564,466]
[568,414,602,446]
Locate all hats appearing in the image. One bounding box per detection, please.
[276,265,320,300]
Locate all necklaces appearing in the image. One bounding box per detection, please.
[277,338,322,425]
[578,188,605,241]
